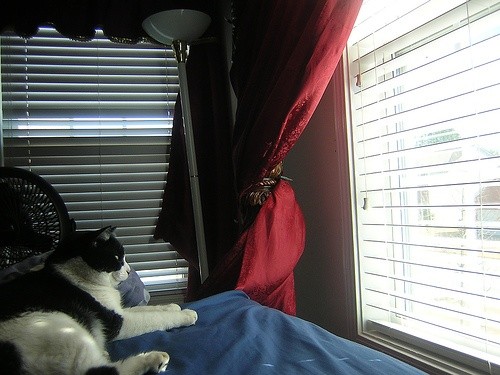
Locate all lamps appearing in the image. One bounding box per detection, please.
[142,9,213,283]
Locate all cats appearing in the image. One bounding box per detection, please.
[0,225,198,374]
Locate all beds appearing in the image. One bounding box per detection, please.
[106,291,431,375]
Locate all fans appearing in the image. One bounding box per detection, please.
[0,167,71,271]
